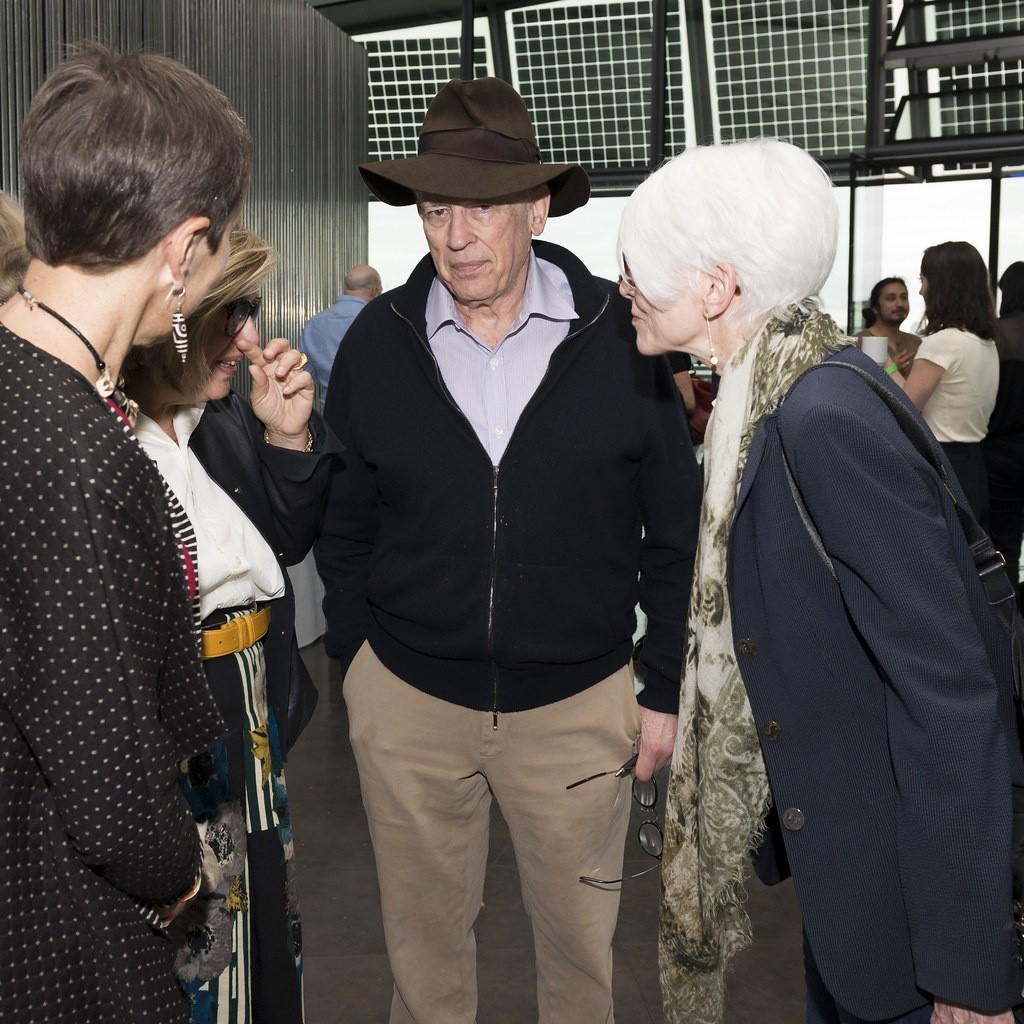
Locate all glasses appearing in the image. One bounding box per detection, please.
[222,296,262,336]
[563,766,666,883]
[621,252,742,298]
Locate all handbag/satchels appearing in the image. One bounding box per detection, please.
[684,374,715,444]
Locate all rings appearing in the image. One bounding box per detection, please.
[290,352,309,370]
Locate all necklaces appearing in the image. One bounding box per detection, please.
[15,282,139,429]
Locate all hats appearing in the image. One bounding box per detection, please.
[356,76,589,217]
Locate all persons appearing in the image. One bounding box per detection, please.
[301,263,386,421]
[611,131,1024,1024]
[881,240,1003,518]
[6,29,258,1021]
[104,210,333,1020]
[301,76,703,1022]
[850,277,924,380]
[666,350,698,416]
[2,186,37,310]
[993,260,1024,455]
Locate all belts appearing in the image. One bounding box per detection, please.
[201,607,273,659]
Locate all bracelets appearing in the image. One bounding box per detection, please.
[883,364,899,376]
[263,427,314,453]
